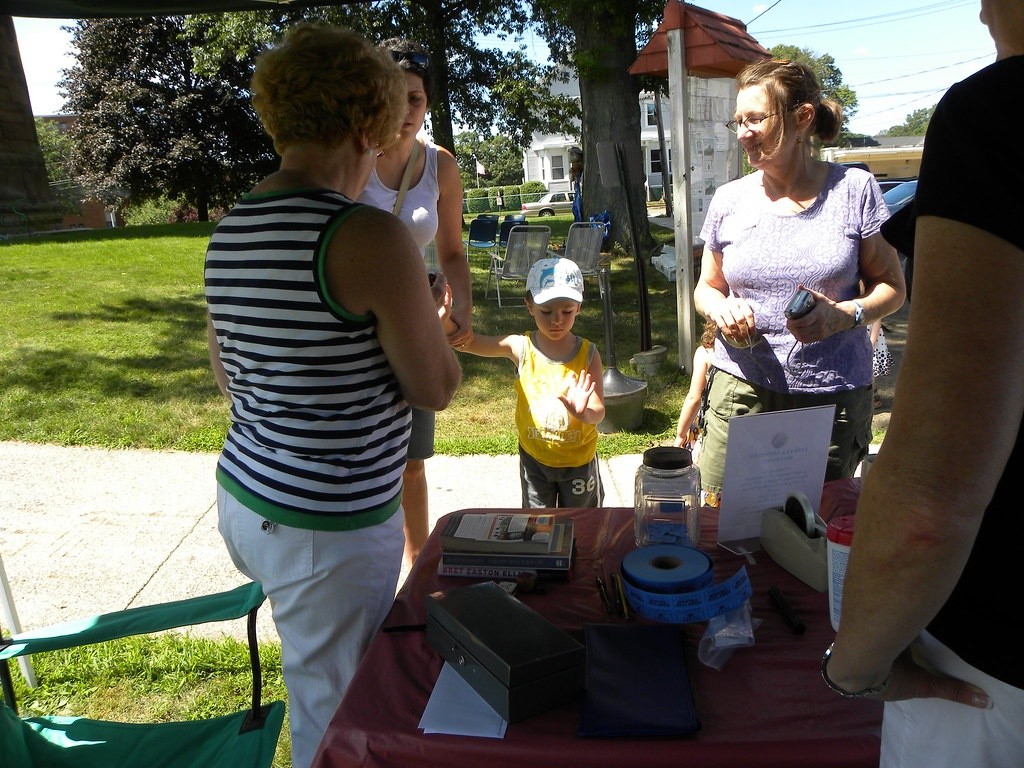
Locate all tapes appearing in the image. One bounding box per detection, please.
[783,491,828,537]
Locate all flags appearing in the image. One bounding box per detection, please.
[477,161,485,174]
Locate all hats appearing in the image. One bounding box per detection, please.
[526,257,584,306]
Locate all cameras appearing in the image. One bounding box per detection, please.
[783,291,815,320]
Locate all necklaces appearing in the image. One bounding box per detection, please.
[439,257,606,510]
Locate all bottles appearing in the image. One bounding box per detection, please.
[633,446,700,548]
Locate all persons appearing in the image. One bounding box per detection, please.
[353,36,475,567]
[203,25,462,768]
[694,58,908,507]
[824,0,1024,768]
[856,272,893,411]
[679,322,717,455]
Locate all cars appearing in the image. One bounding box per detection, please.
[880,180,920,216]
[520,190,579,218]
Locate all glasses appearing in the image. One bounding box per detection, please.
[390,50,429,70]
[725,104,801,133]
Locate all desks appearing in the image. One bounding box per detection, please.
[309,505,885,768]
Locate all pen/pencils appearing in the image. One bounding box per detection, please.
[383,623,427,631]
[612,574,629,619]
[596,577,613,613]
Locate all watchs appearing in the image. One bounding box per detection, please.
[851,301,866,328]
[820,642,889,698]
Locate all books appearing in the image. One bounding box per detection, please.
[437,513,575,579]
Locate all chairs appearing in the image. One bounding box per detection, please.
[0,582,286,768]
[462,212,606,309]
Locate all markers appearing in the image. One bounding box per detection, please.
[767,588,807,634]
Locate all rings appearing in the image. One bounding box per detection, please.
[461,344,464,348]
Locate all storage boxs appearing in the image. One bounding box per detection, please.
[417,581,586,725]
